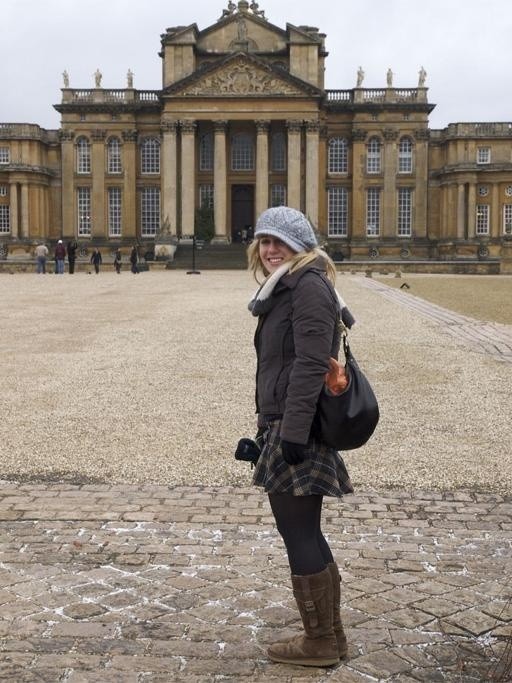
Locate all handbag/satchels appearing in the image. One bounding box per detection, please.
[314,357,379,450]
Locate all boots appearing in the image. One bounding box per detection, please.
[265,563,347,666]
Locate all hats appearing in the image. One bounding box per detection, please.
[254,206,316,252]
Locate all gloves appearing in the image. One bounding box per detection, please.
[281,440,304,466]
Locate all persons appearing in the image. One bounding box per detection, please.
[236,206,354,666]
[67,241,78,274]
[92,68,102,88]
[238,227,254,243]
[62,69,69,88]
[357,66,365,87]
[35,241,49,274]
[130,246,140,274]
[418,66,427,86]
[386,68,393,88]
[114,247,123,274]
[250,0,269,21]
[217,0,236,21]
[55,240,66,274]
[90,247,102,274]
[126,68,137,88]
